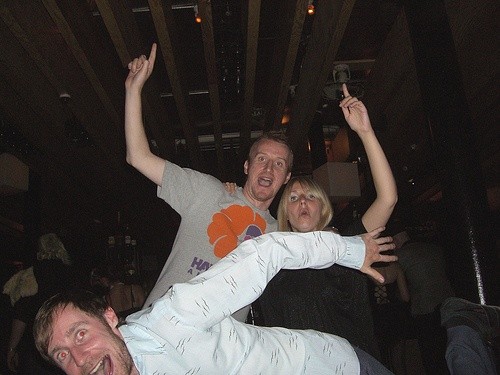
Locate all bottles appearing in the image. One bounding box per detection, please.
[106,235,138,283]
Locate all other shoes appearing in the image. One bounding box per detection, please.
[441,297,500,348]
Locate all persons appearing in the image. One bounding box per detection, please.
[2,232,73,367]
[33,225,399,375]
[393,229,456,351]
[245,82,398,357]
[123,42,294,323]
